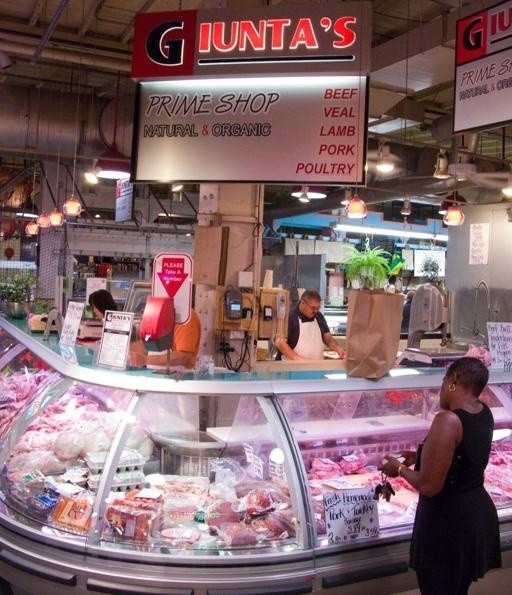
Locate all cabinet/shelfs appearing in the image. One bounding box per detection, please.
[1,305,512,594]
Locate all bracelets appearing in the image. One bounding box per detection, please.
[398,464,407,476]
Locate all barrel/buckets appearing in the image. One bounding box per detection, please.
[224,285,243,319]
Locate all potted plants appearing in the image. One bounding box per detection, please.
[1,272,39,318]
[339,235,395,292]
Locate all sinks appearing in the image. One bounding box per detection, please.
[445,339,481,351]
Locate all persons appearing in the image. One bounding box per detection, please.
[274,290,347,362]
[131,308,202,371]
[88,289,118,324]
[377,357,502,594]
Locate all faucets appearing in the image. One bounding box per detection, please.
[475,280,492,311]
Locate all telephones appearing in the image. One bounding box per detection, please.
[277,295,285,320]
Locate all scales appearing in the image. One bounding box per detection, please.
[76,318,103,340]
[396,347,468,366]
[28,313,59,333]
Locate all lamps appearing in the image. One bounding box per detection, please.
[400,1,412,216]
[433,149,451,179]
[24,0,134,240]
[366,146,403,180]
[345,3,368,218]
[340,190,351,207]
[501,187,512,199]
[438,137,467,226]
[290,185,328,203]
